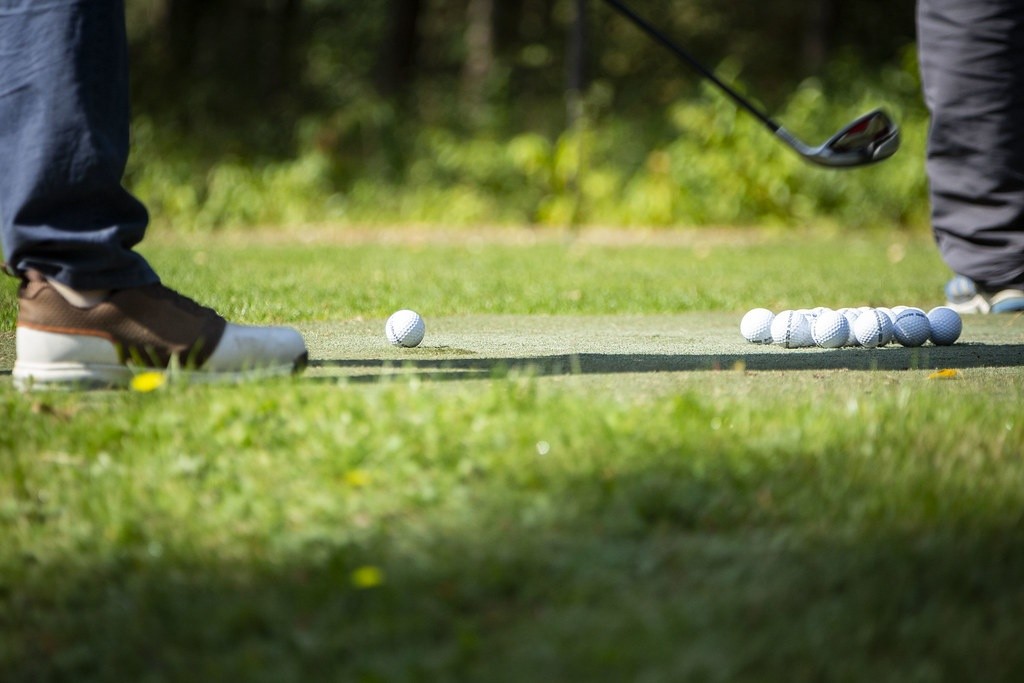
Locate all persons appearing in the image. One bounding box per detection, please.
[918,0,1024,314]
[0,0,308,396]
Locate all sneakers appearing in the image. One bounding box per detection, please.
[946,278,1024,314]
[0,262,308,393]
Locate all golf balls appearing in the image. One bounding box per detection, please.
[386,309,425,348]
[739,304,964,348]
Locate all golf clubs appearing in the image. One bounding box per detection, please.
[608,0,903,168]
